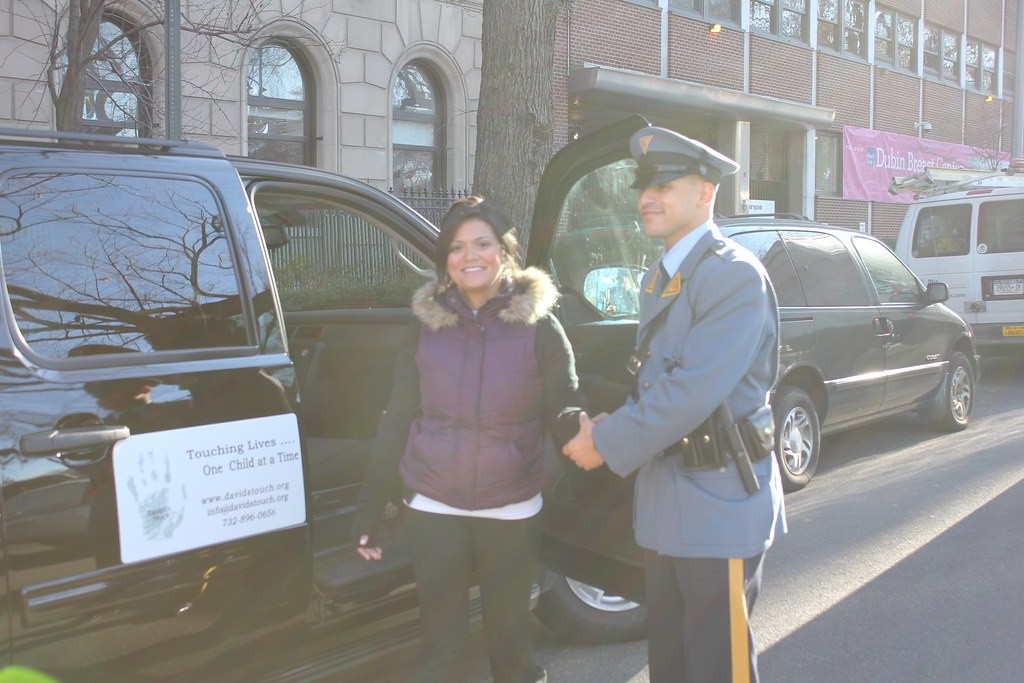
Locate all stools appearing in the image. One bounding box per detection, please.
[305,435,375,493]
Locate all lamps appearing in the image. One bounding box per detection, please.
[876,65,889,74]
[983,95,993,102]
[707,23,721,34]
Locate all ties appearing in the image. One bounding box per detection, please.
[658,259,670,296]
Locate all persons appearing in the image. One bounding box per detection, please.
[562,127,787,683]
[614,269,640,313]
[350,196,587,683]
[90,361,292,574]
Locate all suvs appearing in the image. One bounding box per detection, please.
[0,112,667,683]
[534,212,978,491]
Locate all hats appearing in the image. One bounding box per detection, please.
[629,126,740,192]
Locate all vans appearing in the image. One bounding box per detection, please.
[890,163,1023,359]
